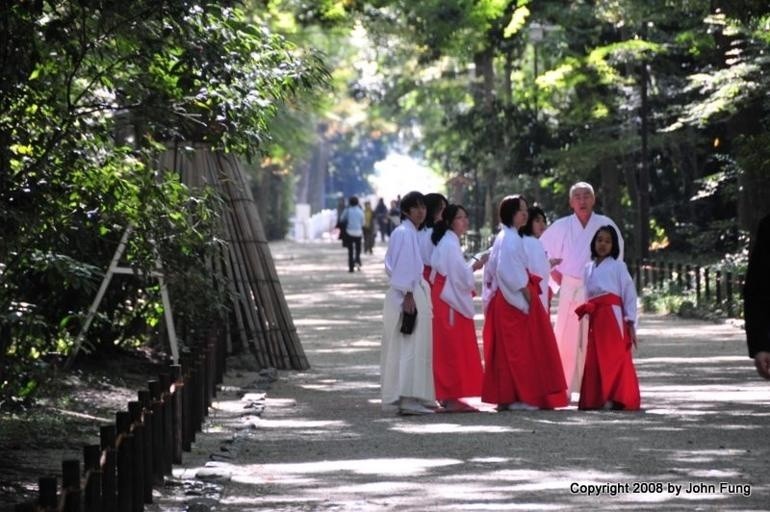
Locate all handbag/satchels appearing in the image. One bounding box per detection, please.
[343,237,350,247]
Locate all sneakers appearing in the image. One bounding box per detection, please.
[604,400,613,409]
[508,402,538,411]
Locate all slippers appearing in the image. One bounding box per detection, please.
[399,405,435,415]
[447,404,478,412]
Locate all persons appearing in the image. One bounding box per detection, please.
[744,216,770,381]
[335,192,402,273]
